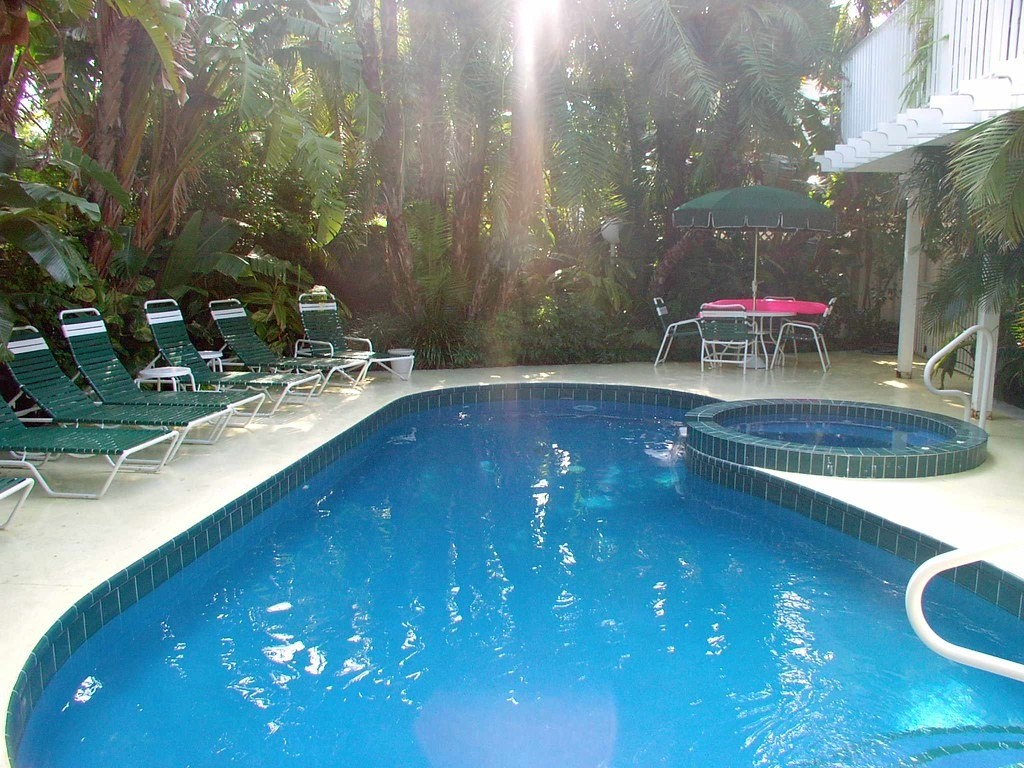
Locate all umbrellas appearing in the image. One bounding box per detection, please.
[673,185,839,355]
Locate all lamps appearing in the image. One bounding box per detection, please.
[600,217,619,266]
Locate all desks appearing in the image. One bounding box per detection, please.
[700,311,798,369]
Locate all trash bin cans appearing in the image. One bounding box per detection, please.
[387,348,415,378]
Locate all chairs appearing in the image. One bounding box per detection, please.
[652,295,838,374]
[0,290,416,535]
